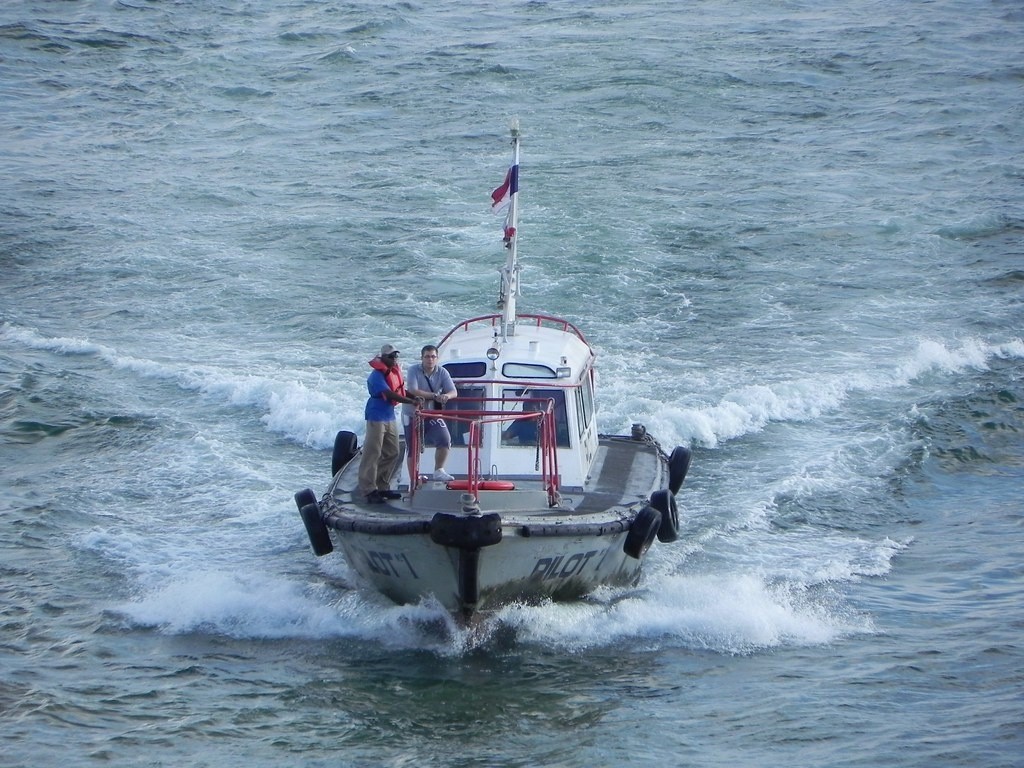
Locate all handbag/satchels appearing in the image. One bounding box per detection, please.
[433,399,447,410]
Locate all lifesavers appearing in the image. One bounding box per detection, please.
[669,446,693,497]
[331,431,358,478]
[294,488,317,511]
[447,479,516,491]
[430,512,503,547]
[650,489,681,543]
[299,502,334,557]
[623,506,663,560]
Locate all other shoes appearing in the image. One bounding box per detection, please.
[364,490,388,504]
[378,490,402,500]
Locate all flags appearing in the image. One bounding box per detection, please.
[491,167,511,215]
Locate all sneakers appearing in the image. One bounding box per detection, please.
[408,479,411,493]
[433,468,454,481]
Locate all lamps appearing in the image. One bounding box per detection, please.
[487,341,502,371]
[556,367,571,378]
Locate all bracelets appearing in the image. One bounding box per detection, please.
[433,394,438,400]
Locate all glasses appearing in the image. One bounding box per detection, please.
[382,354,399,358]
[422,354,438,359]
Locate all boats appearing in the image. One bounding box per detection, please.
[291,111,694,613]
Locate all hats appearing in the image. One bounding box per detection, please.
[380,343,400,355]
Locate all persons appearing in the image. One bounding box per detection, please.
[359,344,424,505]
[401,345,458,482]
[502,395,565,447]
